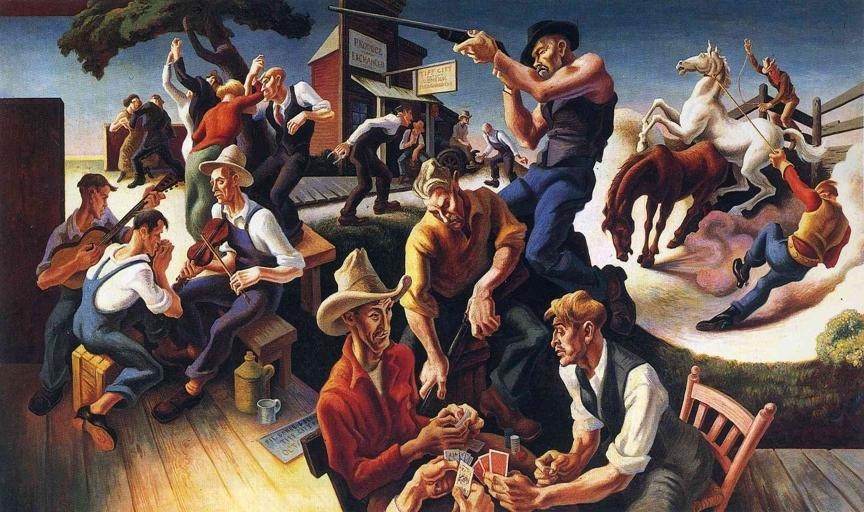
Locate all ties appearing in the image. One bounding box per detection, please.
[274,105,283,125]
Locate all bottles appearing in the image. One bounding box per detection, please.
[232,350,274,416]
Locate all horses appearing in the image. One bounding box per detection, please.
[601,140,729,269]
[635,39,830,216]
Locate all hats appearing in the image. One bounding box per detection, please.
[762,56,776,74]
[318,248,412,336]
[199,144,254,188]
[520,19,579,70]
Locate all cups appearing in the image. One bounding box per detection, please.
[256,398,284,426]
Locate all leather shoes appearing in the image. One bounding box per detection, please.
[603,265,636,337]
[375,200,400,215]
[339,214,368,227]
[484,179,499,187]
[28,386,62,416]
[152,386,202,423]
[128,178,145,189]
[71,405,117,452]
[479,389,542,441]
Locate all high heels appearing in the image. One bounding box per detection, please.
[696,308,732,331]
[734,258,745,289]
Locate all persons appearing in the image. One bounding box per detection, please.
[29,17,855,510]
